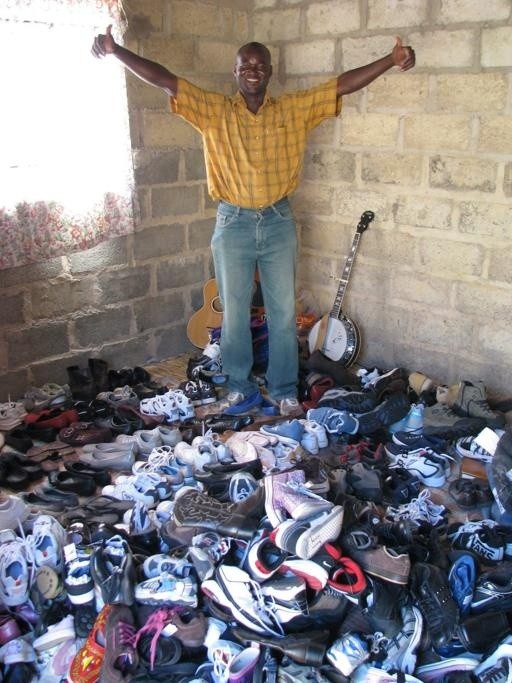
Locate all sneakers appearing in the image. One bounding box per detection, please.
[0,349,512,683]
[201,326,222,359]
[250,317,268,344]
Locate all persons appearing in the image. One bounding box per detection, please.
[88,23,415,421]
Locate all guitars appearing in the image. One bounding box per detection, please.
[187,279,224,350]
